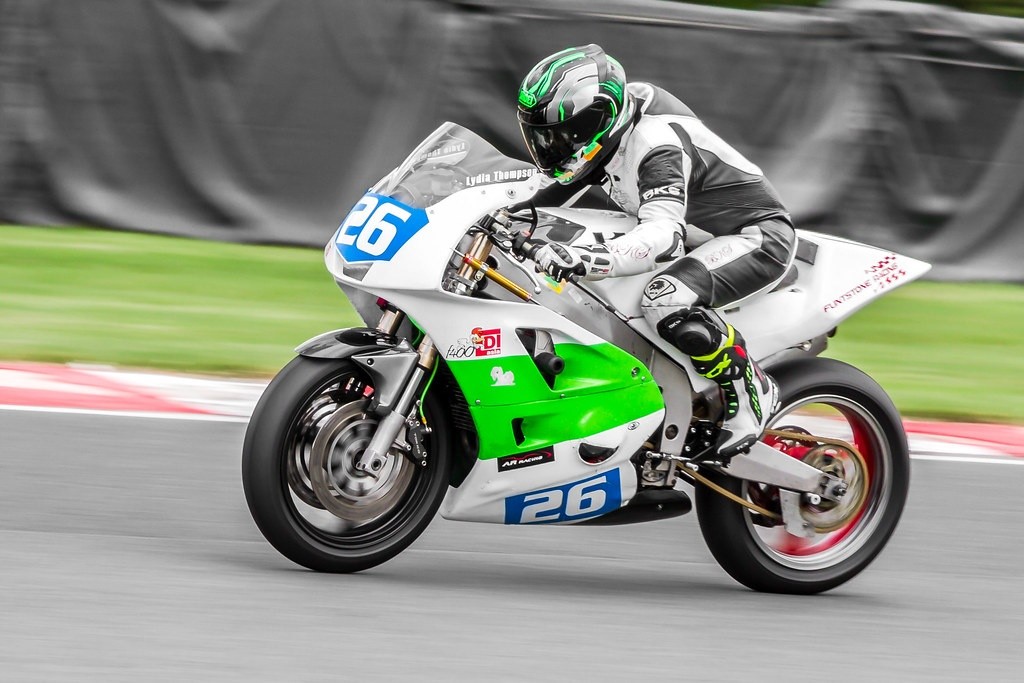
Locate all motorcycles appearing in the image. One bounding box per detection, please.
[242,121,933,595]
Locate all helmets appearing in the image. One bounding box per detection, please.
[517,44,636,186]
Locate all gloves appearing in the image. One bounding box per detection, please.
[535,243,614,282]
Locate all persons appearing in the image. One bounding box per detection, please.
[421,43,798,459]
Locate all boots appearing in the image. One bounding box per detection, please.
[690,323,782,459]
